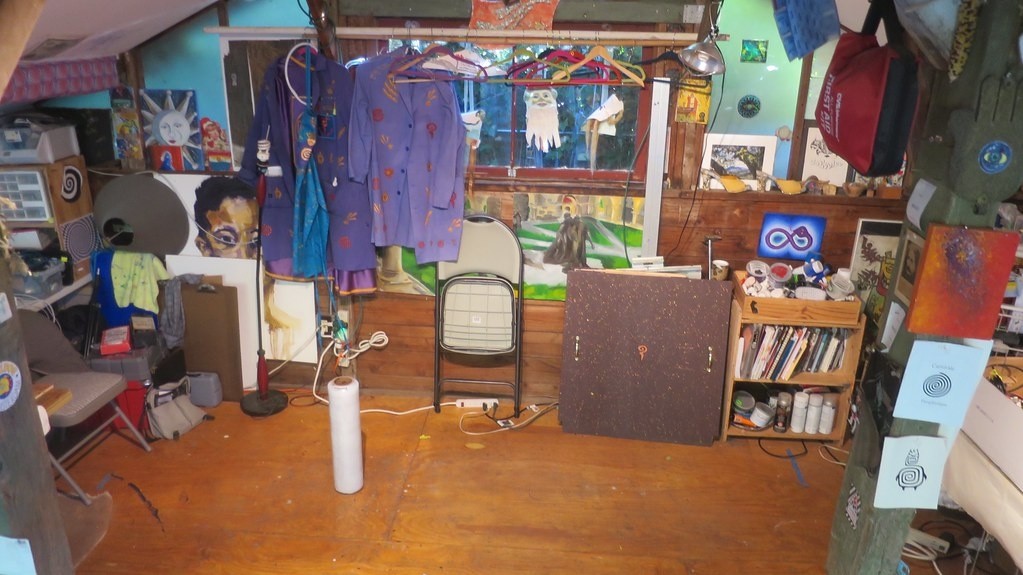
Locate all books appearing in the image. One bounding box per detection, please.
[736,323,847,382]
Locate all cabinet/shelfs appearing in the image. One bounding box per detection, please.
[0,155,102,314]
[720,268,868,449]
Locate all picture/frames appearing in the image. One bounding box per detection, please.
[137,87,205,173]
[697,132,778,192]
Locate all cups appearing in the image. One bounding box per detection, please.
[712,259,730,282]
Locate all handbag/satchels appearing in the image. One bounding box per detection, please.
[814,0,923,183]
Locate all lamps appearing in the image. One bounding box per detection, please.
[676,40,727,77]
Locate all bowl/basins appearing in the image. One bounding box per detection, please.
[843,182,865,198]
[796,287,826,302]
[732,389,756,415]
[745,260,770,283]
[749,402,774,429]
[770,262,793,288]
[825,274,856,300]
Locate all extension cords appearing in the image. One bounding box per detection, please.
[456,398,499,409]
[907,527,950,556]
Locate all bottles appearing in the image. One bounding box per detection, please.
[769,391,840,436]
[791,260,824,280]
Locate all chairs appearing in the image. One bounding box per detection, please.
[433,214,526,420]
[16,308,153,507]
